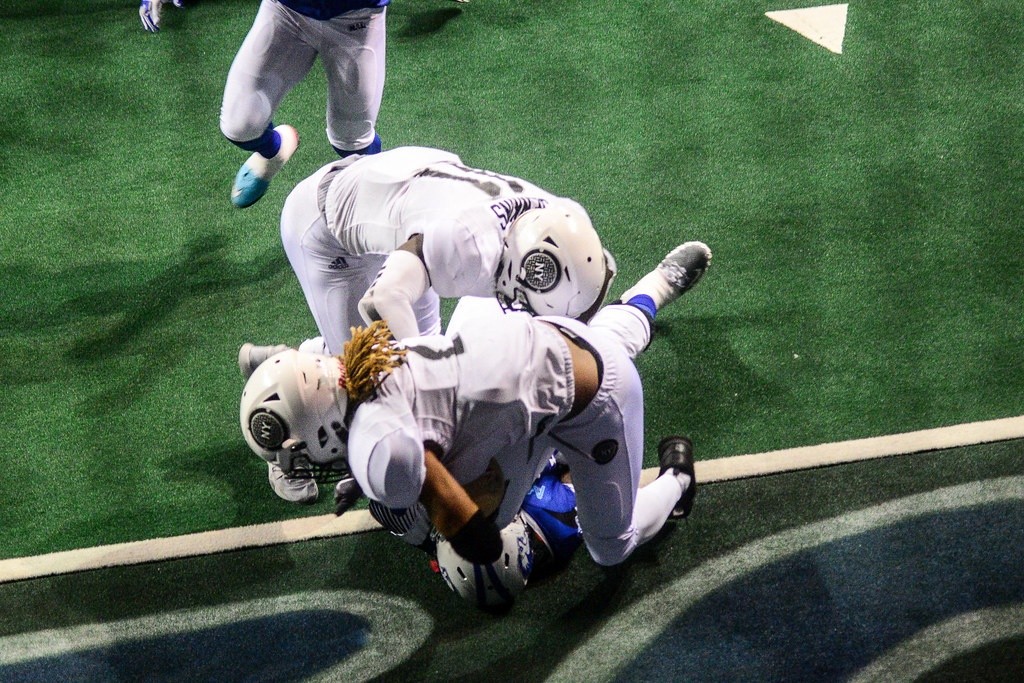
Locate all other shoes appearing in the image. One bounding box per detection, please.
[630,294,656,331]
[619,241,713,316]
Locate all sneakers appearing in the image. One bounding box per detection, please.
[230,124,297,209]
[265,457,319,505]
[657,436,695,521]
[238,344,291,379]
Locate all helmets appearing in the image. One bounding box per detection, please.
[434,507,531,616]
[238,349,352,485]
[497,197,607,324]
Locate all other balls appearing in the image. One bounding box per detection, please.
[430,455,506,520]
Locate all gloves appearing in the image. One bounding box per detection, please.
[140,1,184,35]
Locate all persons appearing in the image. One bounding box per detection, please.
[140,0,390,210]
[235,144,713,608]
